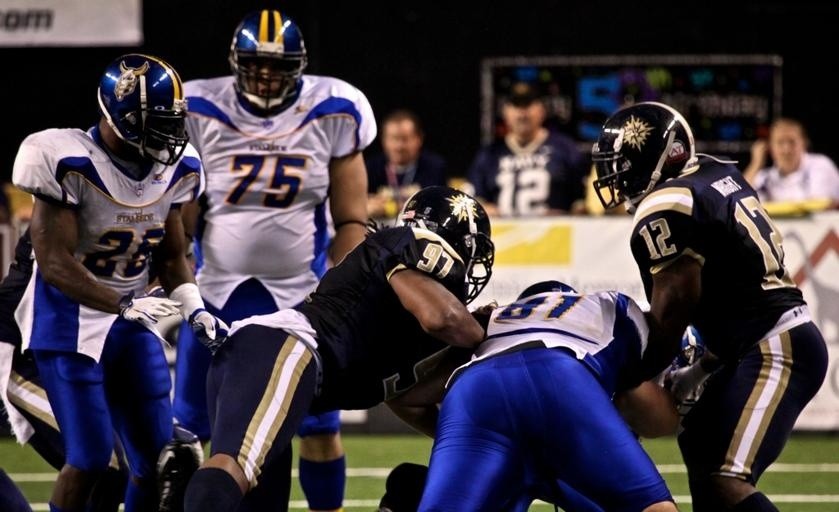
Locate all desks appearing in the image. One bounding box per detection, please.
[326,208,839,432]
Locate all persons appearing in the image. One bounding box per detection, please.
[585,163,634,216]
[15,53,229,512]
[367,114,474,217]
[742,120,839,217]
[406,278,684,512]
[173,9,380,511]
[592,112,828,511]
[475,82,593,217]
[182,183,497,512]
[1,204,204,511]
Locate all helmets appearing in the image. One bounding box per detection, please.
[229,11,307,111]
[394,185,495,307]
[97,54,188,167]
[592,102,697,209]
[518,281,577,298]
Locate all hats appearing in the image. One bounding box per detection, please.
[498,83,549,107]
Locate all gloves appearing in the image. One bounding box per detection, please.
[117,289,183,325]
[662,359,709,406]
[186,307,229,351]
